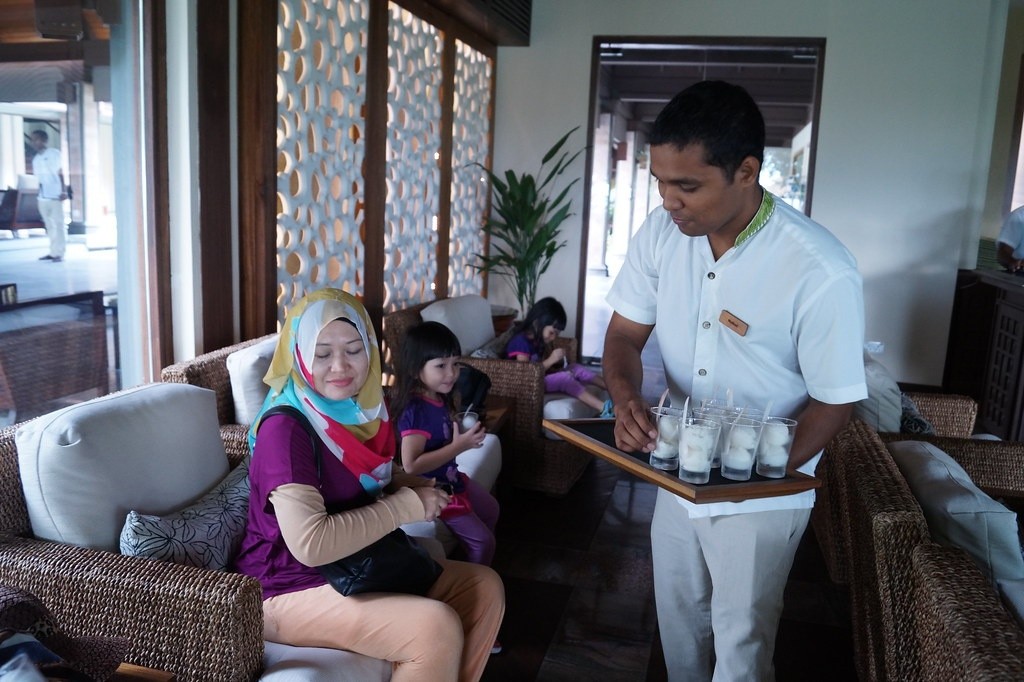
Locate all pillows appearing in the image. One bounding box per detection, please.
[118,453,252,570]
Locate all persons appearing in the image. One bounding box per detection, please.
[602,81,867,682]
[236,288,505,682]
[505,297,615,418]
[393,321,499,567]
[32,130,67,261]
[997,205,1024,274]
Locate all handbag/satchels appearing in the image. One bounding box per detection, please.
[318,496,444,596]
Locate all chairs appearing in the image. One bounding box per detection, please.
[161,332,502,499]
[383,293,604,516]
[0,379,396,682]
[808,386,1024,682]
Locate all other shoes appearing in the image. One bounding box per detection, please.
[599,399,616,418]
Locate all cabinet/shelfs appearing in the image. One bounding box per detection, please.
[939,267,1024,442]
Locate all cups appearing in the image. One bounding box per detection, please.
[451,412,478,435]
[719,417,762,481]
[678,417,722,484]
[648,406,692,471]
[693,397,763,469]
[754,416,798,479]
